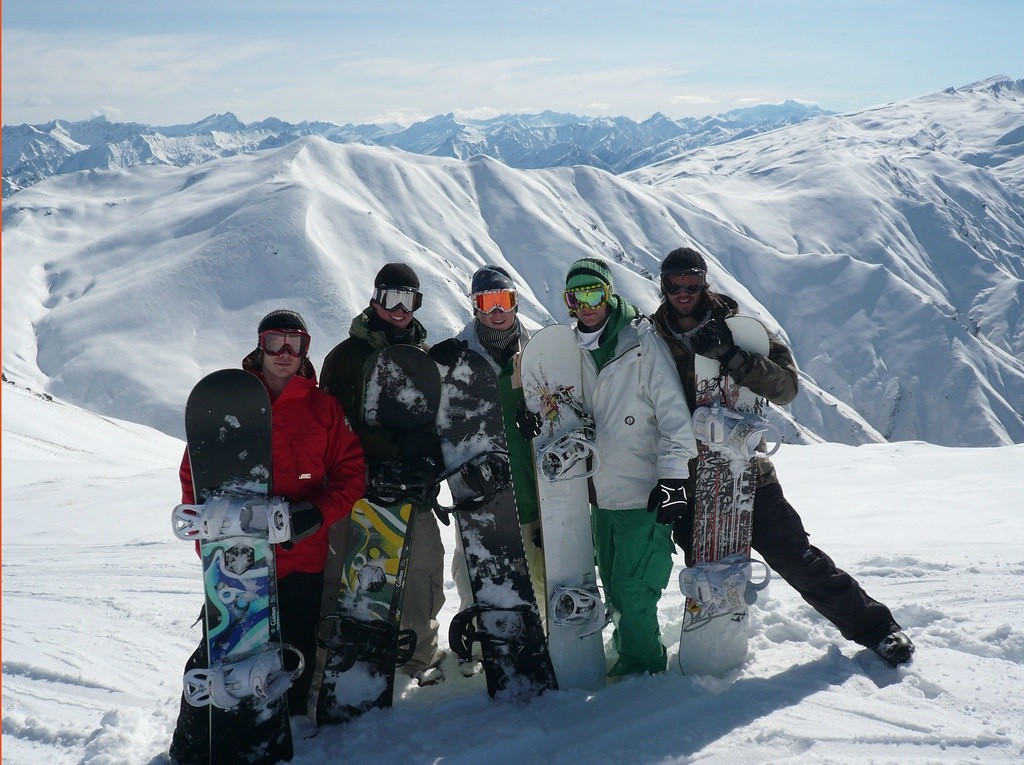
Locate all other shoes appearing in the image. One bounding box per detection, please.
[411,667,444,687]
[459,659,482,678]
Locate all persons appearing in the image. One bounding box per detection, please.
[178,307,366,739]
[514,257,700,682]
[646,248,916,666]
[318,262,545,684]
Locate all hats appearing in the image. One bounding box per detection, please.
[472,265,515,293]
[566,258,614,293]
[664,247,705,274]
[375,262,420,291]
[258,310,307,336]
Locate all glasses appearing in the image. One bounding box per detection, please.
[472,288,517,314]
[563,285,607,313]
[376,288,423,314]
[259,330,311,357]
[661,267,704,293]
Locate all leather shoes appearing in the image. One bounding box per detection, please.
[873,623,913,665]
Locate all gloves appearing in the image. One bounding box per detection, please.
[647,478,689,525]
[514,400,543,440]
[691,317,736,364]
[277,502,321,550]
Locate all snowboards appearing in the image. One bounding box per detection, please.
[679,316,772,676]
[521,324,609,692]
[183,368,306,765]
[433,348,560,704]
[316,343,443,729]
